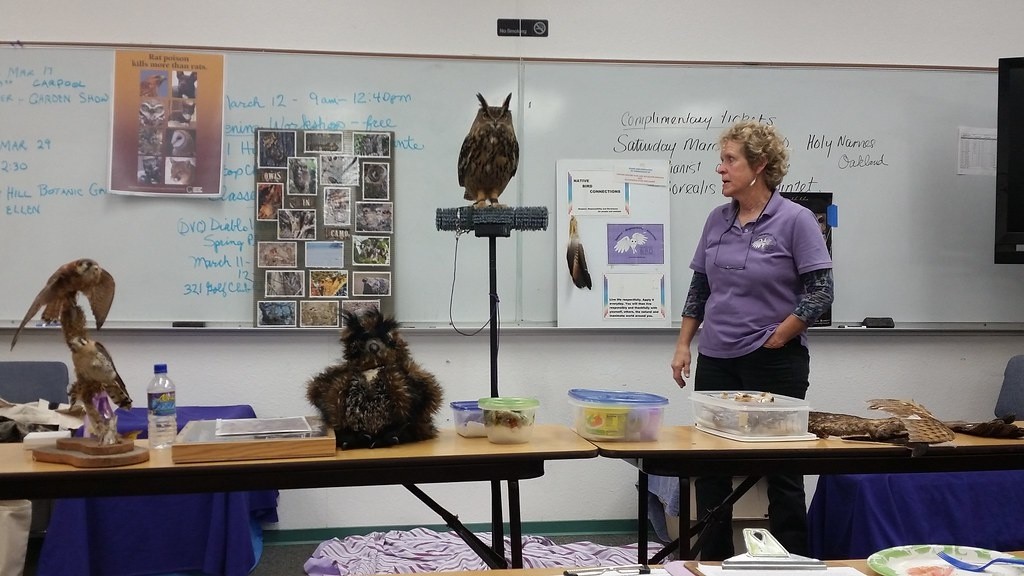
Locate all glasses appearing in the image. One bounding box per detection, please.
[713,229,753,270]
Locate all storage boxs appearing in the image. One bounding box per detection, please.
[478,397,540,443]
[688,390,809,436]
[567,388,669,441]
[450,401,487,437]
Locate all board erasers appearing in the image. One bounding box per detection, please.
[171,319,206,327]
[864,317,894,328]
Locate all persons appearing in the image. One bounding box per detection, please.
[670,122,835,562]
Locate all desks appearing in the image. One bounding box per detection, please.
[0,422,598,576]
[569,421,1024,565]
[36,406,279,576]
[806,470,1024,560]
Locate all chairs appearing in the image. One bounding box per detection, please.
[0,362,69,403]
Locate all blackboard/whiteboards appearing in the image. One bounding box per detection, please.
[0,46,1023,337]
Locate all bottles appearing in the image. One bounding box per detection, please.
[146,364,177,448]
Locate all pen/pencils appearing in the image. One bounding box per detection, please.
[838,324,862,328]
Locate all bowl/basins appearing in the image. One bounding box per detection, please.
[477,396,540,443]
[566,388,669,442]
[450,401,488,438]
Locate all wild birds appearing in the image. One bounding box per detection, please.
[9,259,132,443]
